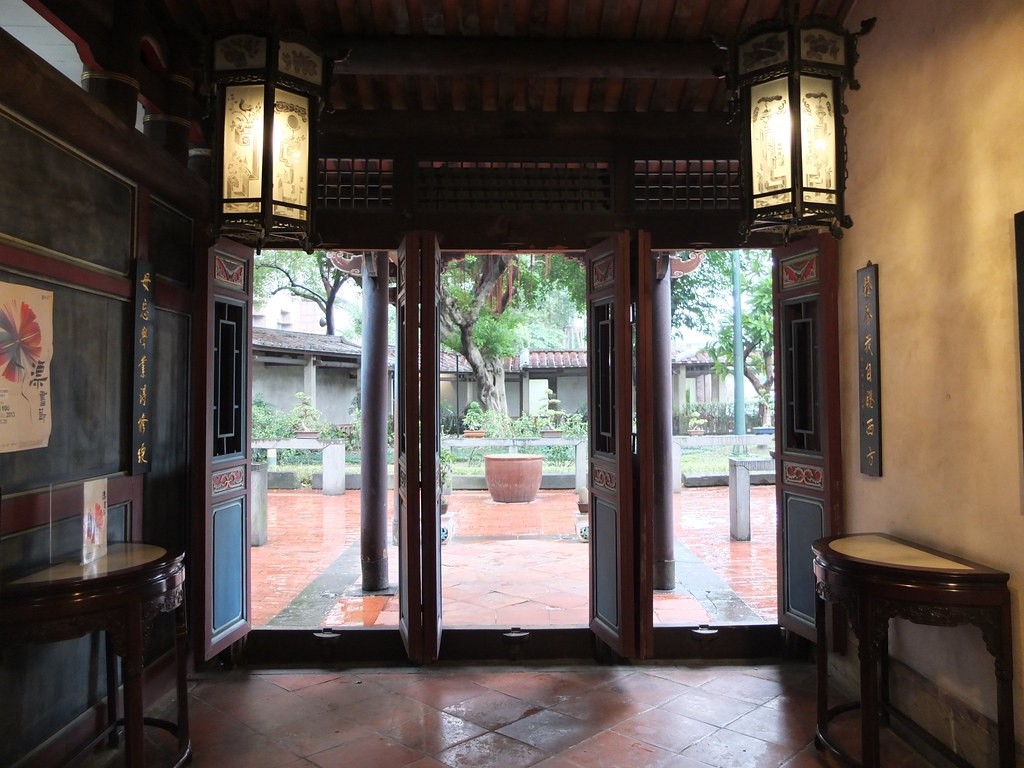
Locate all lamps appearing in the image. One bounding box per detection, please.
[184,0,355,255]
[707,0,879,245]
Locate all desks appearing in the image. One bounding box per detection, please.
[251,438,345,495]
[728,457,775,542]
[0,541,193,768]
[809,531,1013,768]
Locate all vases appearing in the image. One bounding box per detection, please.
[484,454,543,502]
[577,501,588,513]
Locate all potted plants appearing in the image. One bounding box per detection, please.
[752,389,775,435]
[294,391,322,438]
[537,388,566,438]
[461,401,486,437]
[440,448,456,515]
[687,411,708,436]
